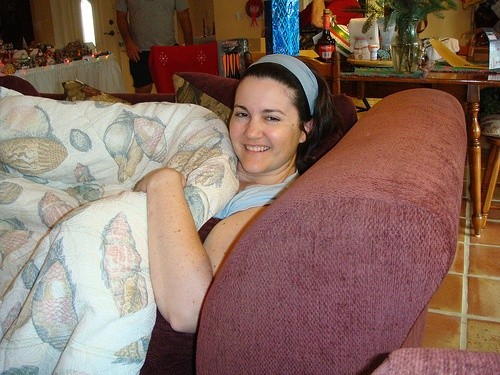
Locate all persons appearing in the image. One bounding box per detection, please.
[133,54,339,375]
[115,0,193,93]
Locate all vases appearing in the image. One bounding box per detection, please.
[390,18,423,73]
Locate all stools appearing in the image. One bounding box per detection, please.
[471,114,500,230]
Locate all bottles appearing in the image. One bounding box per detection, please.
[316,8,336,63]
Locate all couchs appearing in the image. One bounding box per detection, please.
[0,74,466,374]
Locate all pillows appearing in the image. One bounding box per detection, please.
[172,70,357,166]
[61,79,133,106]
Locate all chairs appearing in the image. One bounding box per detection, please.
[243,50,340,96]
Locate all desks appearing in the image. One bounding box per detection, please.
[14,52,126,93]
[343,70,500,236]
